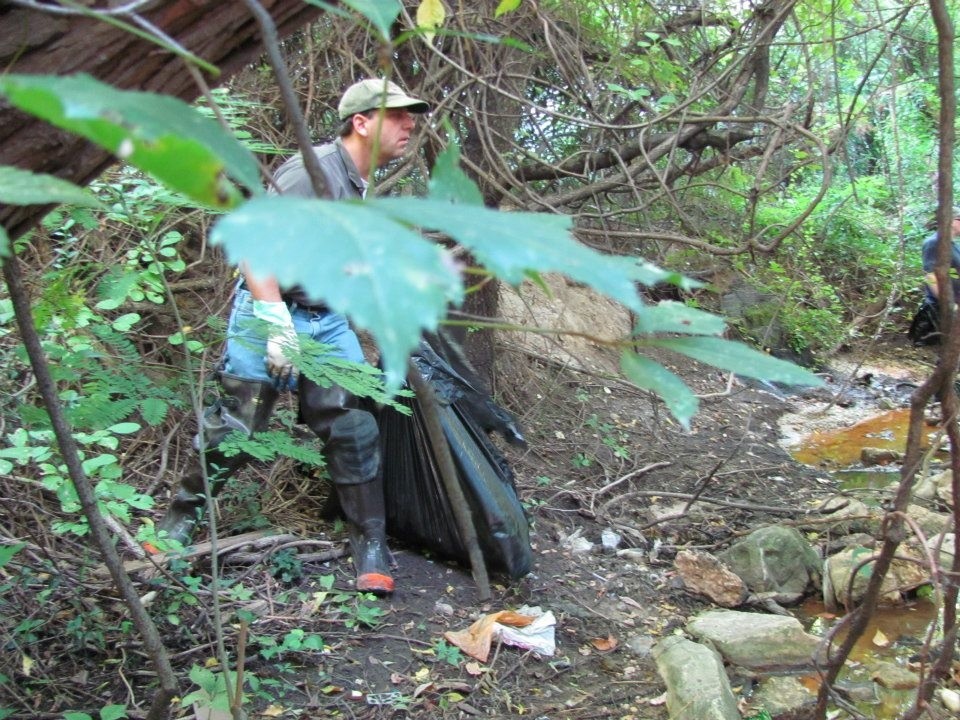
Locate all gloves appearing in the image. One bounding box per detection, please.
[253,300,300,392]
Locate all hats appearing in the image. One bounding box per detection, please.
[338,78,430,120]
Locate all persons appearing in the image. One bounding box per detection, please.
[143,77,432,596]
[906,205,960,347]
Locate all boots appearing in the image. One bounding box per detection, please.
[141,370,279,554]
[299,373,395,592]
